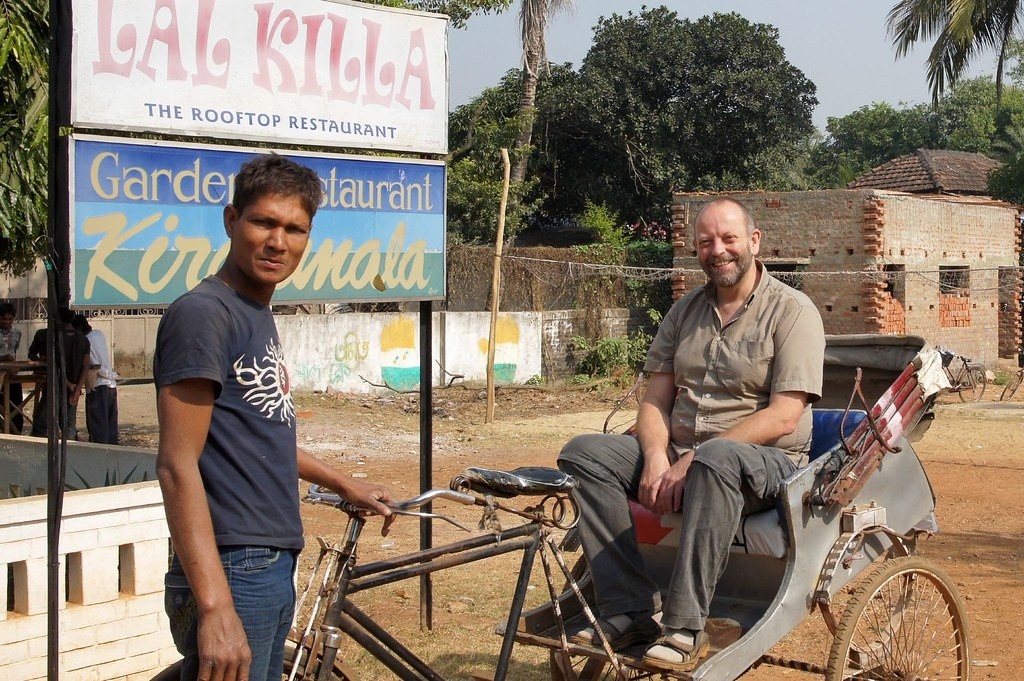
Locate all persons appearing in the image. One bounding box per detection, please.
[559,199,825,671]
[152,156,398,681]
[0,302,24,437]
[28,306,118,445]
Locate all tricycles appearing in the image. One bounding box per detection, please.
[150,335,972,681]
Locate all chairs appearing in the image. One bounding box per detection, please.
[627,409,864,598]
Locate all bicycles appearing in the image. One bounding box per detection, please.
[934,346,987,403]
[1000,346,1024,401]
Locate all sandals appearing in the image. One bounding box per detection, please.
[569,610,661,651]
[641,628,710,672]
